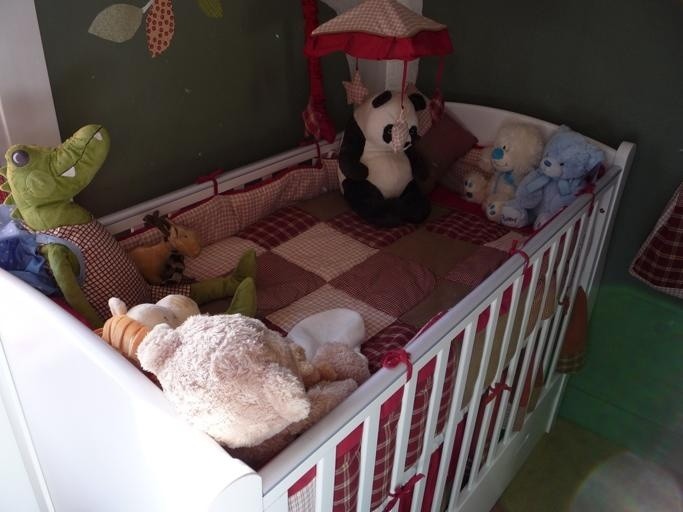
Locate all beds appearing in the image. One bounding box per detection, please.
[0,103,637,511]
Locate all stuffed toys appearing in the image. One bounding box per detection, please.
[499,123,605,232]
[461,122,544,223]
[1,122,260,327]
[135,314,371,469]
[101,292,201,393]
[128,208,203,285]
[335,89,433,228]
